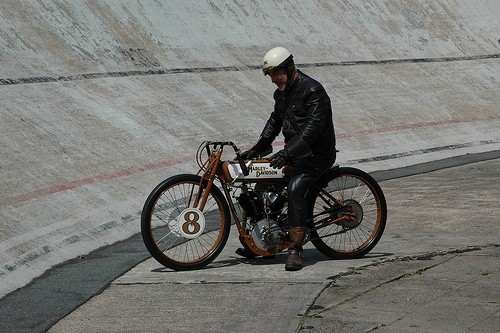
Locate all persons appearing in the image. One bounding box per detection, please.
[232,46,336,271]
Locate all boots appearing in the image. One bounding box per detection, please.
[284,226,307,270]
[236,248,276,259]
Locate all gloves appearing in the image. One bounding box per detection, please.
[269,135,313,170]
[234,137,274,161]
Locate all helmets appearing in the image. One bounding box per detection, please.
[263,47,292,76]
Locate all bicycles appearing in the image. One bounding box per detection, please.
[141,141,388,270]
